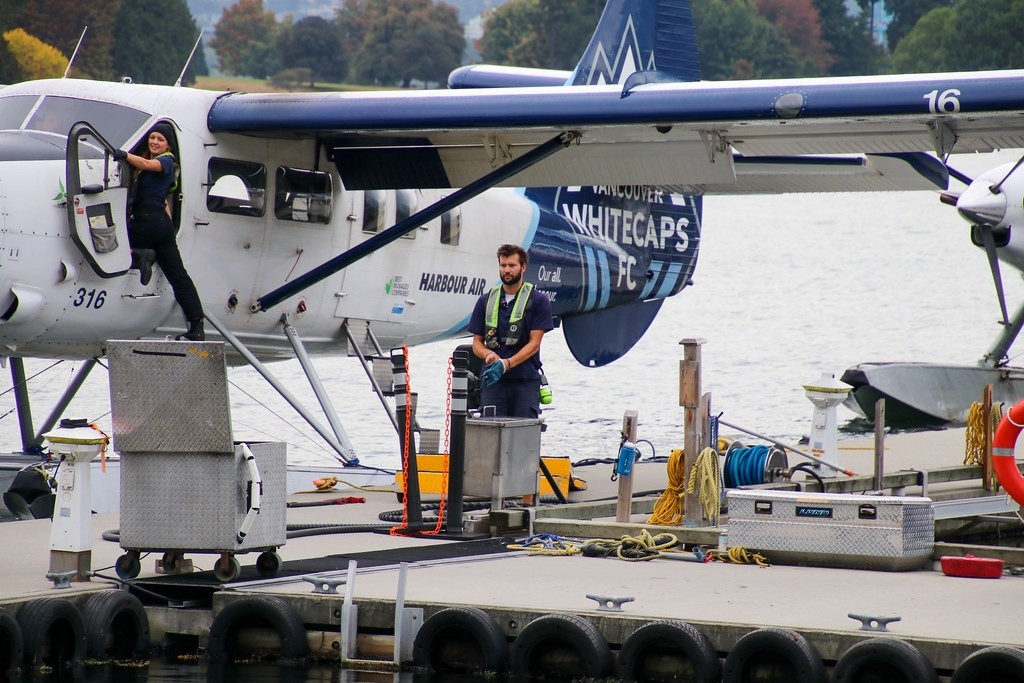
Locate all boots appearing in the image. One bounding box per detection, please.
[176,322,207,342]
[132,247,155,285]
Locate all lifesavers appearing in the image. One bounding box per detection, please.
[207,596,305,657]
[616,620,722,683]
[412,672,504,683]
[830,637,939,683]
[950,647,1024,683]
[0,611,23,669]
[508,615,610,679]
[89,665,149,683]
[23,600,87,665]
[205,658,308,683]
[412,607,506,671]
[992,400,1024,507]
[29,666,85,683]
[723,628,826,683]
[88,591,150,660]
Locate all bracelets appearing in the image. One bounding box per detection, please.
[484,351,495,357]
[506,358,511,370]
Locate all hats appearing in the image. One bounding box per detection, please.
[149,122,174,149]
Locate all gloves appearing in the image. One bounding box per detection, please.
[113,149,127,161]
[480,359,506,388]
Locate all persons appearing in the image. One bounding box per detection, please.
[113,126,206,341]
[466,244,555,419]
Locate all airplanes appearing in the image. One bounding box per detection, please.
[1,1,1024,521]
